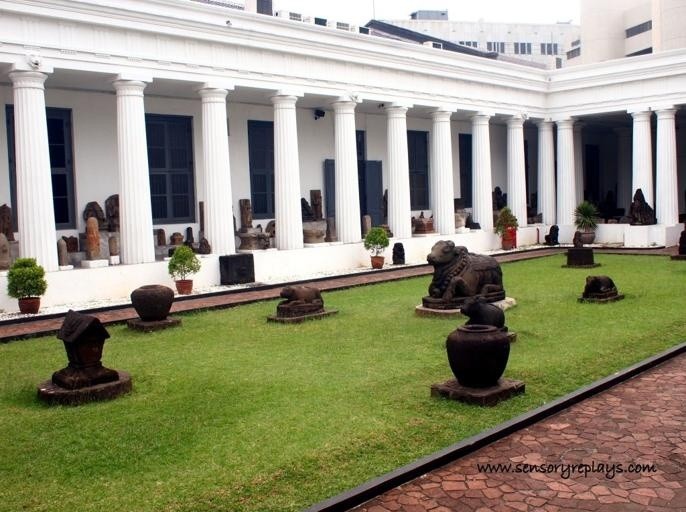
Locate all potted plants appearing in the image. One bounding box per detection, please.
[167,245,201,295]
[493,206,520,250]
[5,256,48,313]
[364,226,389,270]
[573,200,601,246]
